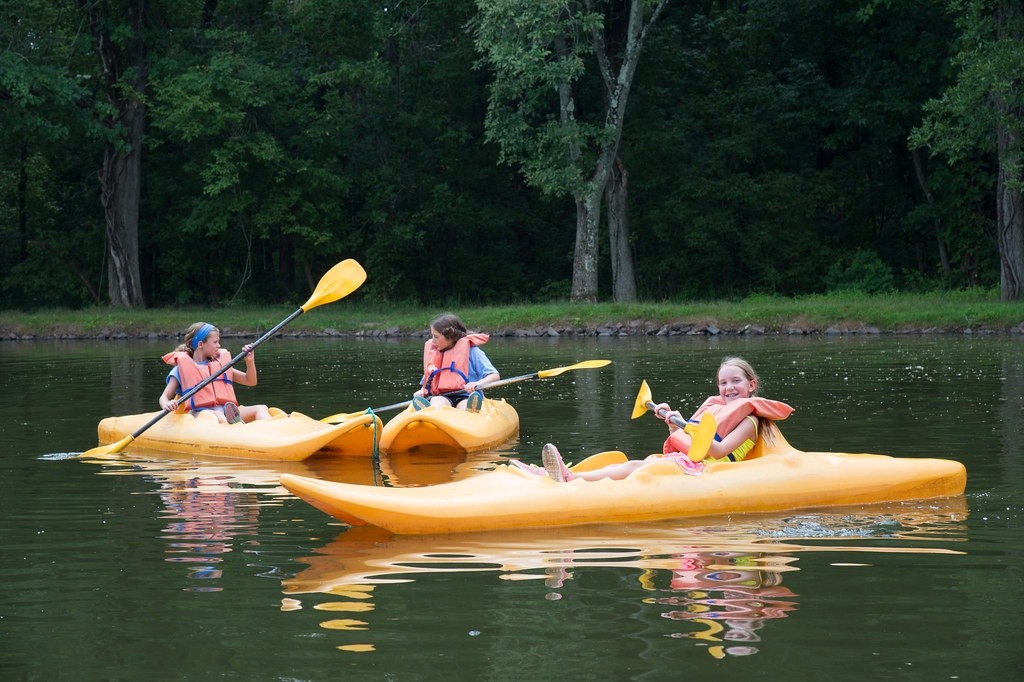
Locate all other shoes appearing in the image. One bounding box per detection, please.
[465,392,484,414]
[223,403,245,425]
[412,396,432,410]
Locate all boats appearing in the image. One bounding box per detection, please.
[97,398,524,462]
[280,452,969,537]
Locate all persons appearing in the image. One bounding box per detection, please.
[508,358,770,482]
[413,315,500,414]
[159,322,272,426]
[158,473,803,659]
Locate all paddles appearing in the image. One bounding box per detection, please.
[630,379,720,463]
[318,356,613,428]
[74,258,368,454]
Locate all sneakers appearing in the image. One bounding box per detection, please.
[542,443,574,482]
[509,458,546,478]
